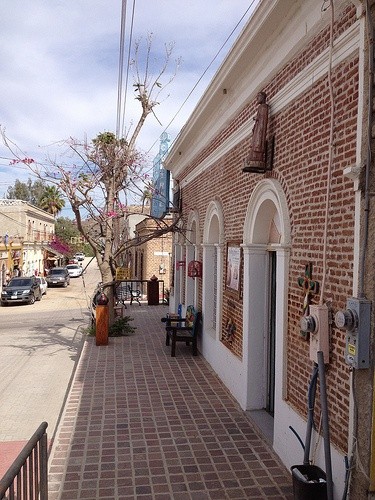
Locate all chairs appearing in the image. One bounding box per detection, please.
[164,304,201,356]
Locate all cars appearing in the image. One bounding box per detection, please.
[65,264,82,277]
[2,275,48,306]
[47,268,70,289]
[68,253,85,264]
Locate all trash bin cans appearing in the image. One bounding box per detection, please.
[290,464,334,500]
